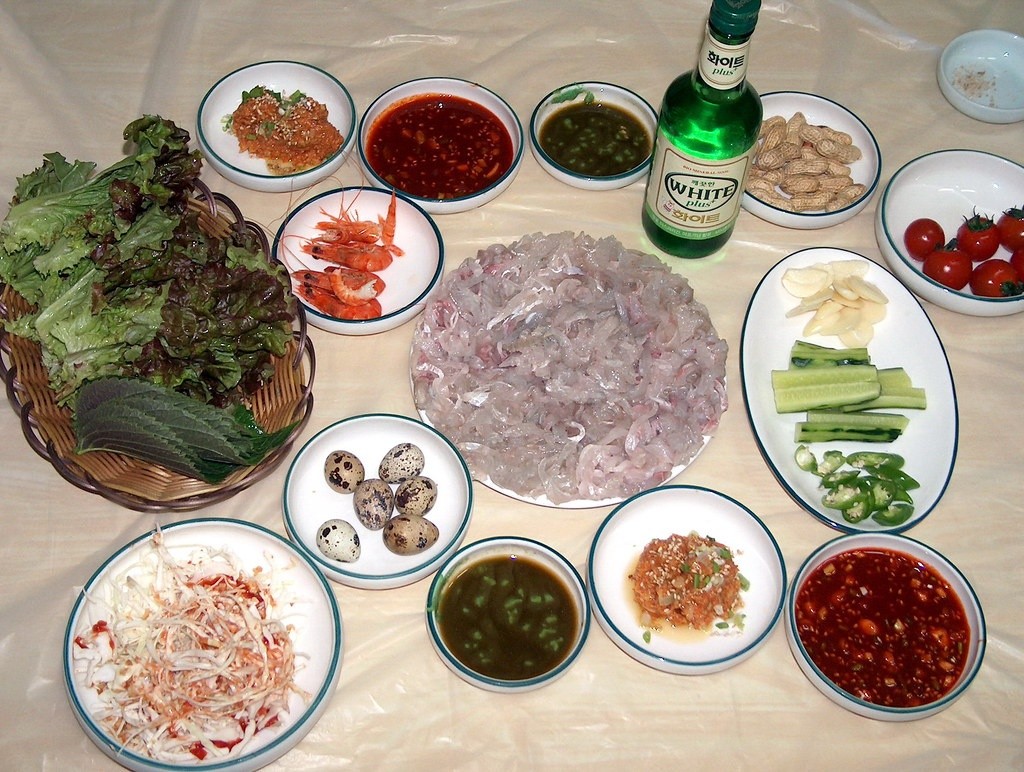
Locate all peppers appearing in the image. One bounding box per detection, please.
[795,444,920,526]
[548,107,648,181]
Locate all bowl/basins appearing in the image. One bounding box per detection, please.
[280,412,473,590]
[62,517,345,772]
[353,76,524,215]
[528,80,659,191]
[195,59,358,193]
[785,532,988,721]
[874,148,1024,316]
[271,186,444,335]
[584,484,788,677]
[427,536,592,694]
[741,90,882,230]
[936,28,1024,124]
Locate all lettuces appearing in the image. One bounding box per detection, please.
[0,113,296,410]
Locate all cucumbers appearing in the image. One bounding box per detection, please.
[770,340,927,443]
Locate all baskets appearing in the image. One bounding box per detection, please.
[0,176,318,514]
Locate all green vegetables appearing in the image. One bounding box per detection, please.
[64,374,302,485]
[222,85,334,170]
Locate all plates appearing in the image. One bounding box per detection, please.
[410,331,728,508]
[738,247,959,535]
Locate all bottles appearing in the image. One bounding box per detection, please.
[640,0,763,261]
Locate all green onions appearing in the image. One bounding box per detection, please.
[643,536,751,643]
[453,564,564,667]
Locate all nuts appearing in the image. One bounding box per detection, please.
[745,112,868,213]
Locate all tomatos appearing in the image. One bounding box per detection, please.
[904,207,1024,298]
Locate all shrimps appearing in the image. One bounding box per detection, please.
[263,146,404,321]
